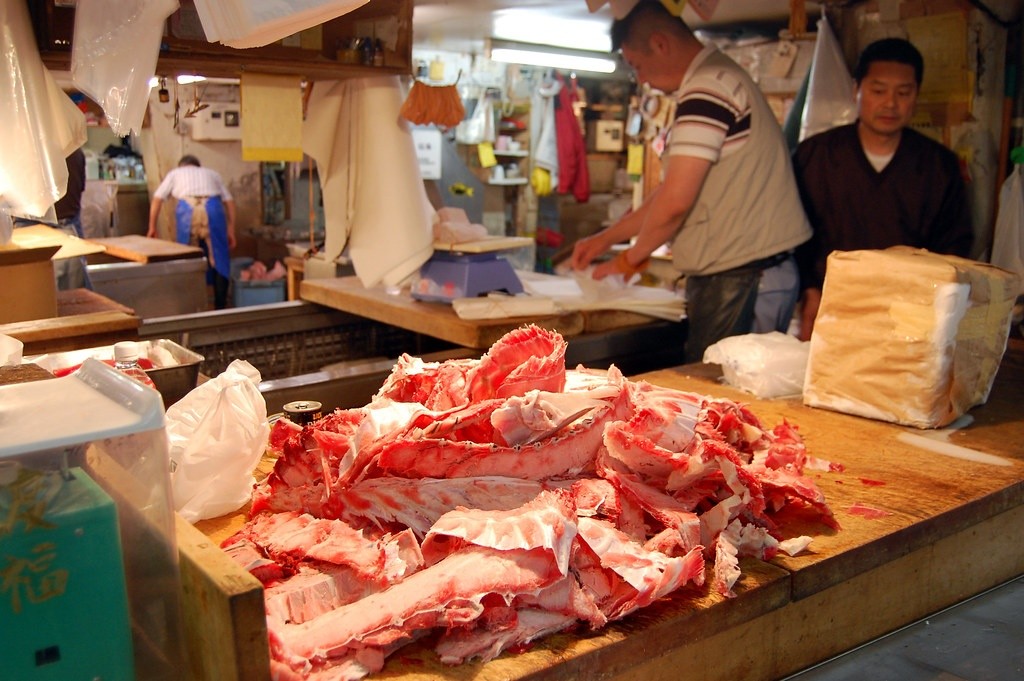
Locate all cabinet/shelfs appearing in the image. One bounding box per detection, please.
[482,147,530,187]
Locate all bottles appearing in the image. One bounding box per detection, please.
[362,37,373,64]
[114,341,156,390]
[586,104,611,151]
[373,39,384,63]
[607,105,625,153]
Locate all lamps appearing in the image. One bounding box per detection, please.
[482,37,620,74]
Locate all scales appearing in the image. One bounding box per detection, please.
[410,236,534,303]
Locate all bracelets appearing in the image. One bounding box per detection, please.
[619,250,650,273]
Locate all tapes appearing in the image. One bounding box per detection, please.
[336,49,362,64]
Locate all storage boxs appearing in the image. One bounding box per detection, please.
[804,244,1024,431]
[227,256,286,308]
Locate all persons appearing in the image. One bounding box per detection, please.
[146,154,237,309]
[104,134,143,159]
[571,0,816,363]
[53,148,95,292]
[787,41,976,340]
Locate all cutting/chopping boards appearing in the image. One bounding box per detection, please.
[300,270,657,349]
[195,362,1024,681]
[85,234,204,264]
